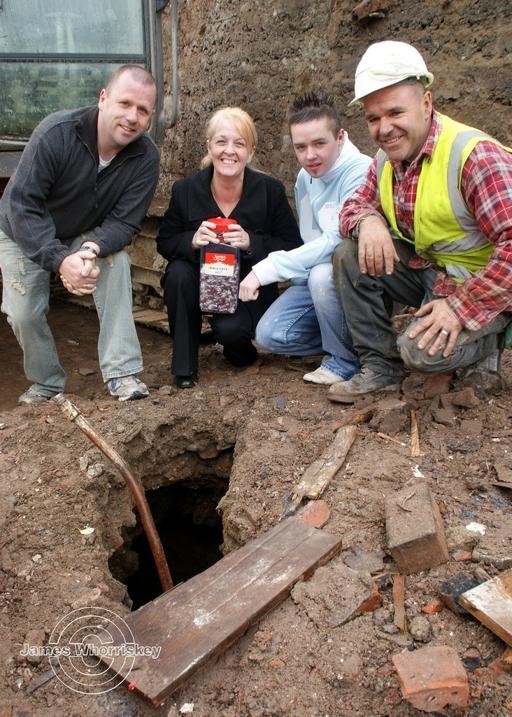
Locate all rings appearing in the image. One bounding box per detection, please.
[441,330,448,337]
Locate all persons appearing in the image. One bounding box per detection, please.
[238,91,374,385]
[0,65,159,405]
[326,41,512,404]
[156,107,303,388]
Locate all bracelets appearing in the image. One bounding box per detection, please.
[78,246,98,256]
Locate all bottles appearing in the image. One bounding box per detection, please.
[199,219,240,314]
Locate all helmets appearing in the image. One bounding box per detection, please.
[346,40,435,107]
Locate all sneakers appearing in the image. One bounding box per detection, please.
[302,365,344,385]
[454,333,505,400]
[17,388,48,407]
[327,366,403,404]
[108,374,149,401]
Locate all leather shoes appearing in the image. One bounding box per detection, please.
[224,341,258,367]
[176,375,194,389]
